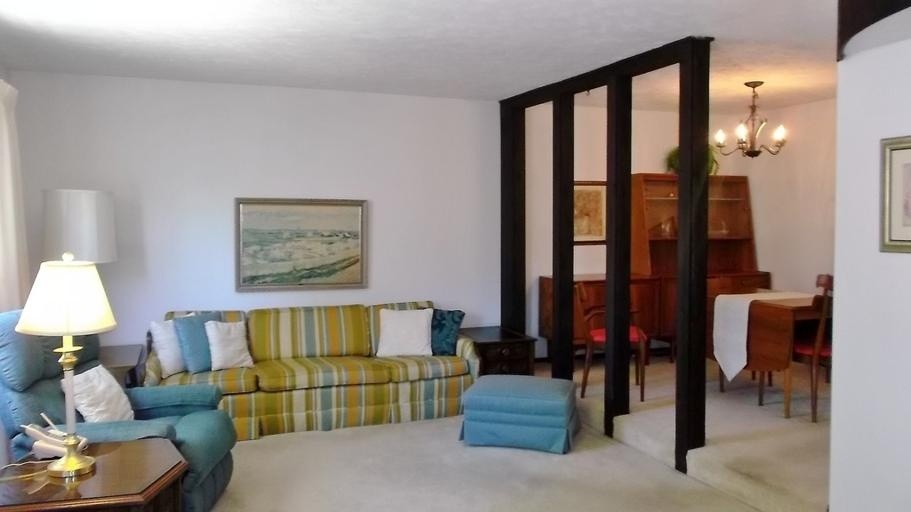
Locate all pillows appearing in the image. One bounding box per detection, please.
[146,312,195,379]
[172,311,221,375]
[60,364,134,423]
[376,307,434,357]
[416,306,466,356]
[203,320,256,370]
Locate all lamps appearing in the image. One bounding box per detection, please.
[714,80,788,159]
[13,250,119,478]
[40,188,118,264]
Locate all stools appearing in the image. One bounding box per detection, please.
[457,374,581,454]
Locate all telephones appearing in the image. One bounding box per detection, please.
[20,413,89,461]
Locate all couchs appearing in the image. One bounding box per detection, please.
[137,298,479,436]
[0,308,237,512]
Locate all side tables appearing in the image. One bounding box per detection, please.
[0,439,190,512]
[461,324,539,376]
[100,344,143,388]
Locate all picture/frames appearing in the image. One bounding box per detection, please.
[233,197,368,293]
[879,134,911,254]
[573,180,606,245]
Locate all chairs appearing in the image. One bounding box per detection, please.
[572,283,647,403]
[758,273,831,421]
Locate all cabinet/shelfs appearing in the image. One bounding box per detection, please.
[538,272,661,358]
[632,172,771,356]
